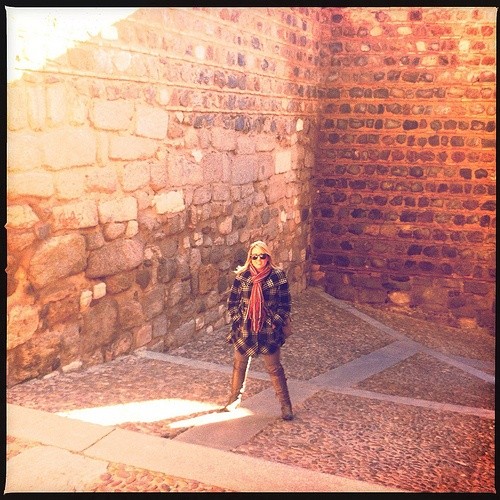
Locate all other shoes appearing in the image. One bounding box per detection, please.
[281,406,293,420]
[224,399,242,412]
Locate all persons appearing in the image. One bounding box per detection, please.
[225,241,293,420]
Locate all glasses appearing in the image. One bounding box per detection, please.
[250,253,268,260]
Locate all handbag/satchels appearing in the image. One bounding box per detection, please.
[282,314,295,338]
